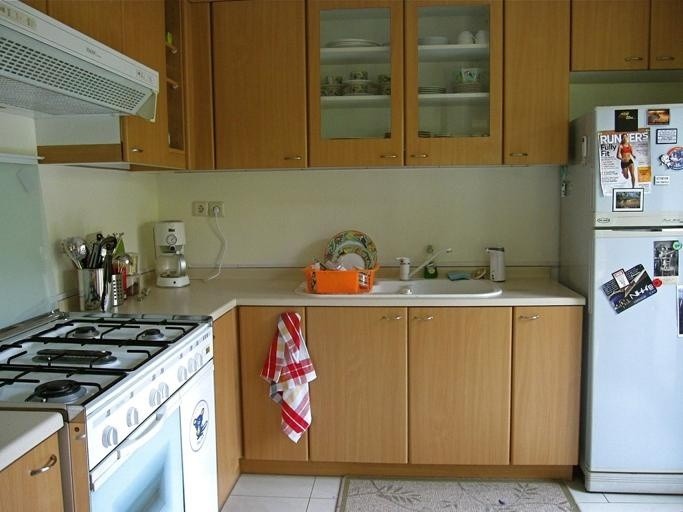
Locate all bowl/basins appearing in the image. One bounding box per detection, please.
[458,30,475,44]
[472,30,488,46]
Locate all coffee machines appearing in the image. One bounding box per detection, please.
[154,220,190,287]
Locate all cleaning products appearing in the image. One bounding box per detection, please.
[424,245,439,279]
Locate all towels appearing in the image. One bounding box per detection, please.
[260,310,316,442]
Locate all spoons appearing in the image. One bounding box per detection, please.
[115,254,131,277]
[63,237,86,269]
[100,237,116,264]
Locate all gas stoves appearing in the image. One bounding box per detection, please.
[0,313,217,410]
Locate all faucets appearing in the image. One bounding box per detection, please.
[395,247,452,280]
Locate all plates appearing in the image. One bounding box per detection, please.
[327,38,382,49]
[416,86,447,94]
[419,37,449,44]
[313,229,378,287]
[345,78,372,88]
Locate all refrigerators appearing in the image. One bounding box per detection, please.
[557,104,683,494]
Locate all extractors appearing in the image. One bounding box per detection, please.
[0,1,151,122]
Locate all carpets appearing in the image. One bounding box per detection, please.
[333,474,580,512]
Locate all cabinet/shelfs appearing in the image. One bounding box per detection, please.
[569,0,682,70]
[510,306,583,480]
[0,431,64,512]
[503,0,570,166]
[212,307,242,512]
[211,0,309,170]
[305,307,512,480]
[239,306,309,474]
[304,0,503,168]
[19,0,187,173]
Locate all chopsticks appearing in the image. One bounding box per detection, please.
[83,241,98,269]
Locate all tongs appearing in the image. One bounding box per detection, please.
[99,256,114,314]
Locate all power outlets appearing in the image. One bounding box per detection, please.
[193,200,208,217]
[208,201,224,218]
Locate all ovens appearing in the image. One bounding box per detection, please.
[86,324,218,511]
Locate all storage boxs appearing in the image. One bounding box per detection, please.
[300,263,379,294]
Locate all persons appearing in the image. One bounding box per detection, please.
[616,133,636,189]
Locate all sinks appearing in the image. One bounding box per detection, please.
[294,278,502,297]
